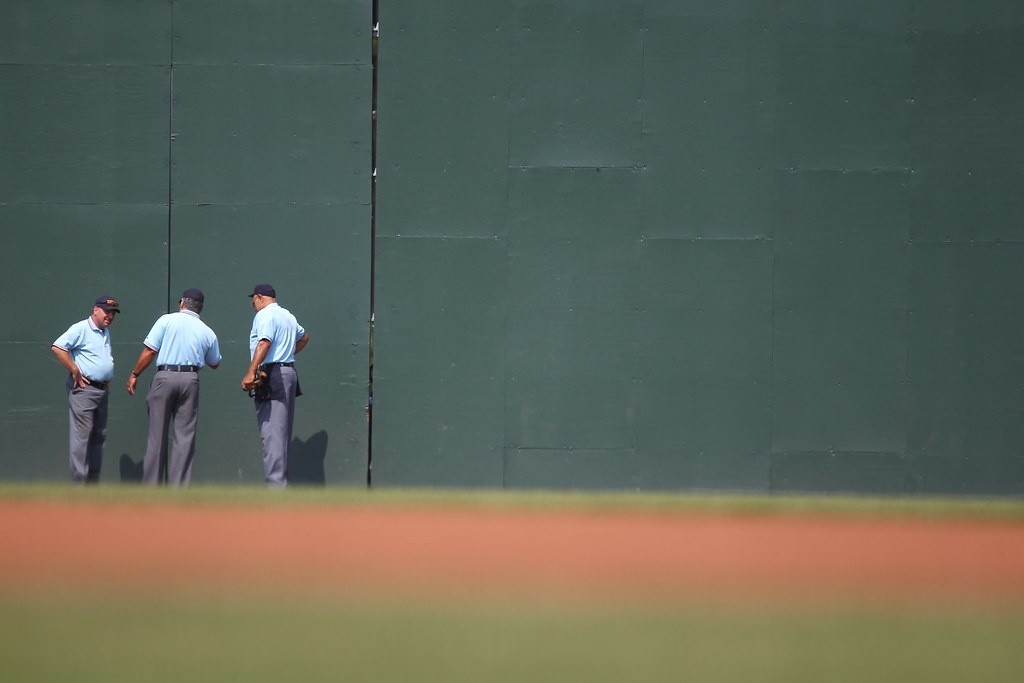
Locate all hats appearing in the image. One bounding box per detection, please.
[247,284,278,299]
[179,289,204,304]
[95,296,121,313]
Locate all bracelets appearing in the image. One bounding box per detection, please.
[131,370,138,377]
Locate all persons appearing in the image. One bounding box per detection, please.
[128,289,223,491]
[51,293,120,486]
[241,283,308,489]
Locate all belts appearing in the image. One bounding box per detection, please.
[82,378,109,390]
[157,364,200,373]
[257,362,293,367]
